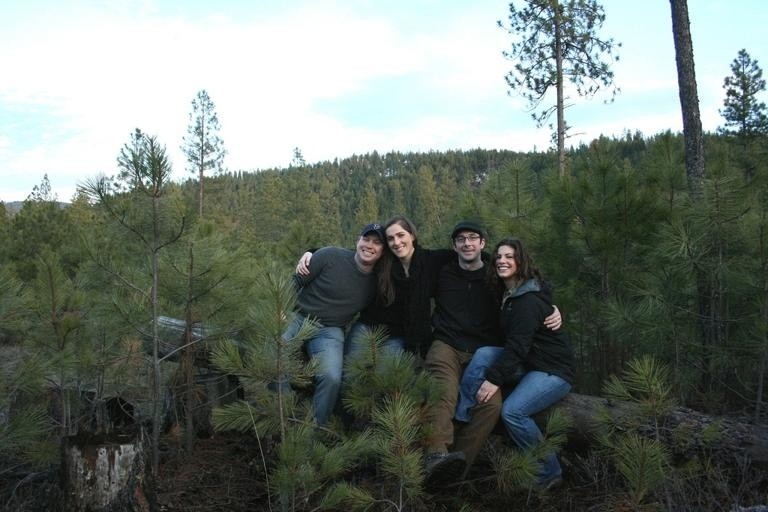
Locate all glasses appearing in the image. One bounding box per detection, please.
[454,234,481,242]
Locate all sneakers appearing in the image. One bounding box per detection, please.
[424,451,467,495]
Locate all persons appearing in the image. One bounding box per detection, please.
[294,216,492,390]
[277,223,386,427]
[421,222,562,511]
[453,238,577,492]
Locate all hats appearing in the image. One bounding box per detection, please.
[361,223,385,242]
[452,222,484,237]
